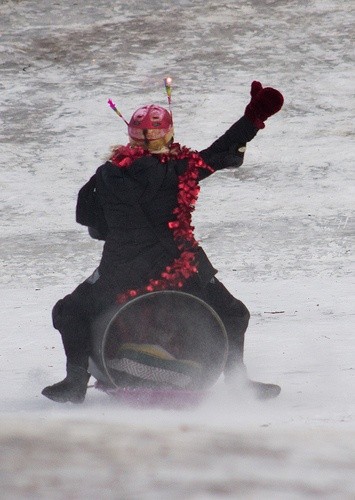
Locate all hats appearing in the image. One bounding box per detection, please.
[128,105,174,149]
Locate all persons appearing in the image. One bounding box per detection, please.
[40,79,284,404]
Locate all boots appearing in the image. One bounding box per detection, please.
[223,364,281,403]
[41,362,91,404]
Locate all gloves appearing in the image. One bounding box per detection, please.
[244,81,284,121]
